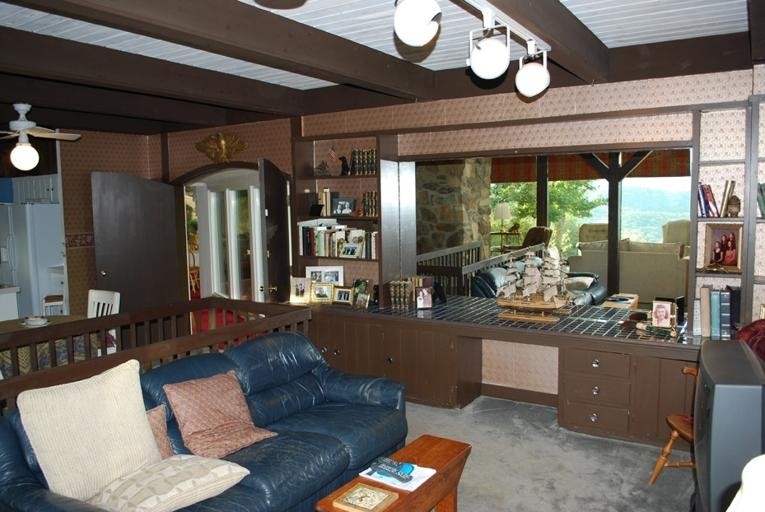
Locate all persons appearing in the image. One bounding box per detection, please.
[709,231,737,266]
[315,287,327,298]
[295,283,304,296]
[339,291,349,300]
[653,304,669,327]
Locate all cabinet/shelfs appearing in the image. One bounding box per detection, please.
[285,315,482,410]
[290,131,399,309]
[564,347,632,436]
[688,95,765,338]
[656,356,699,453]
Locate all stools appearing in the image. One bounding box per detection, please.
[43,295,64,316]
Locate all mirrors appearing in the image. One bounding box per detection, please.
[414,147,692,313]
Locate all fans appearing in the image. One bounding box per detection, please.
[0,103,82,142]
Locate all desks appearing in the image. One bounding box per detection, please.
[1,315,114,381]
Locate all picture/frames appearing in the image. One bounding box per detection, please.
[704,223,743,273]
[339,241,363,259]
[290,265,370,310]
[332,197,354,216]
[651,301,672,328]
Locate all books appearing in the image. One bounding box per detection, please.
[300,224,379,259]
[697,181,736,218]
[390,276,434,311]
[695,283,735,337]
[317,187,331,217]
[351,148,376,176]
[362,191,377,218]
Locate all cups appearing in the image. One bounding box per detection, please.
[25,318,46,325]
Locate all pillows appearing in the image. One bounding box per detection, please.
[85,453,252,511]
[162,368,279,458]
[146,402,175,458]
[16,358,163,500]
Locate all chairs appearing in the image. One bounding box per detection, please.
[87,289,120,356]
[578,223,610,241]
[662,220,691,256]
[648,367,696,487]
[503,226,552,258]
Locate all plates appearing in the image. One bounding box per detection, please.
[604,296,634,302]
[21,323,49,328]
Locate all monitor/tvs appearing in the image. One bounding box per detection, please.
[693,341,764,511]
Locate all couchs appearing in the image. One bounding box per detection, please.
[1,330,407,512]
[568,238,691,306]
[472,256,607,306]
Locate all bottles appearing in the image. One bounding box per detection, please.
[653,327,678,338]
[622,320,651,332]
[681,312,688,335]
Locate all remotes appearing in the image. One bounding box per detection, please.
[372,462,412,483]
[608,296,628,301]
[378,456,401,467]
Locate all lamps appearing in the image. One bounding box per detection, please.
[10,131,39,171]
[395,0,553,98]
